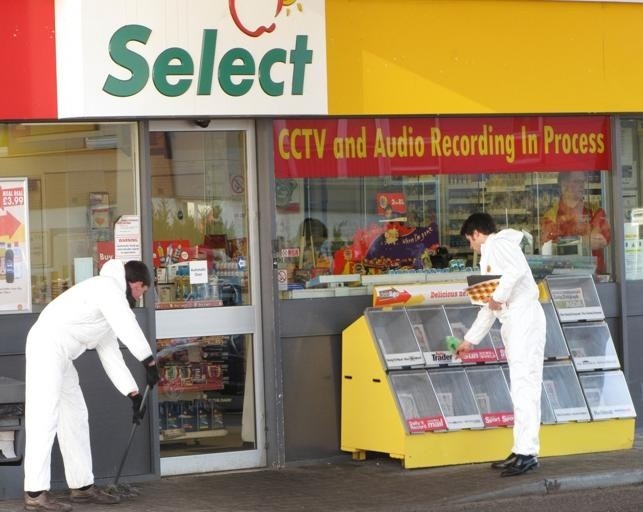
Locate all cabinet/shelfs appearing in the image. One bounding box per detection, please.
[175,268,221,303]
[448,175,484,248]
[445,335,462,356]
[157,241,182,267]
[212,255,247,279]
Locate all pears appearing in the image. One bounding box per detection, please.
[159,399,225,433]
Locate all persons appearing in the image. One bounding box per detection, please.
[526,209,537,225]
[21,261,160,510]
[540,170,612,272]
[452,213,548,477]
[289,217,328,263]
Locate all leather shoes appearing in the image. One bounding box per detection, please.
[144,364,161,389]
[128,393,147,424]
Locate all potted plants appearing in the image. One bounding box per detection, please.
[0,176,32,314]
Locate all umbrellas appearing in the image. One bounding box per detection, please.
[69,483,121,505]
[501,454,538,477]
[23,489,73,512]
[492,451,517,468]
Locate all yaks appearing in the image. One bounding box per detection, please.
[103,384,150,499]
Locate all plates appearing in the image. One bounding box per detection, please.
[402,171,602,254]
